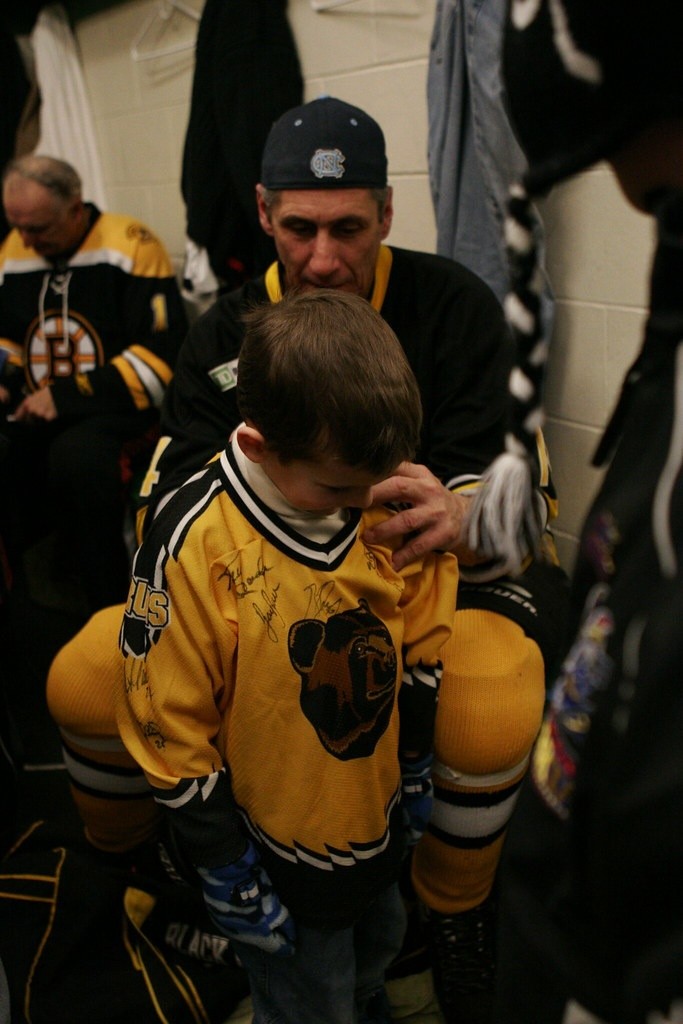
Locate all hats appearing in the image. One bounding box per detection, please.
[260,97,389,189]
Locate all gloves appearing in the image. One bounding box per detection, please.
[195,841,297,956]
[394,751,433,859]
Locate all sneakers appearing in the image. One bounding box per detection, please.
[420,897,497,1024]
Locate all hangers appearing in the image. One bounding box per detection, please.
[128,0,202,63]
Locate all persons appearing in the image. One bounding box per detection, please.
[0,97,546,1024]
[500,0,683,1024]
